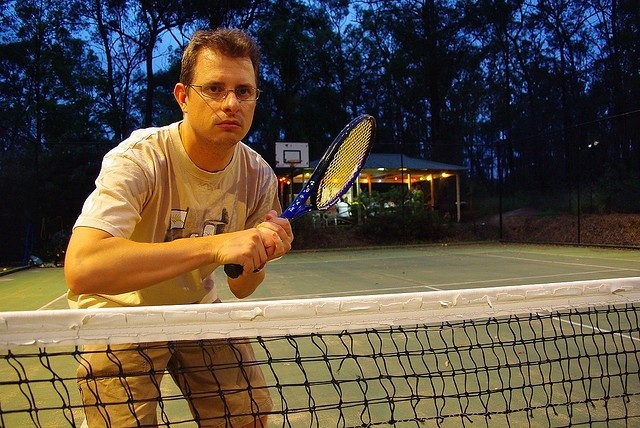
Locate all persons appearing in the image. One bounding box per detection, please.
[414,184,424,211]
[63,28,295,428]
[337,198,350,224]
[411,182,417,203]
[328,205,337,220]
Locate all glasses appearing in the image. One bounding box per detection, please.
[189,83,262,101]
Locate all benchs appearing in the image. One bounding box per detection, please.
[331,217,353,224]
